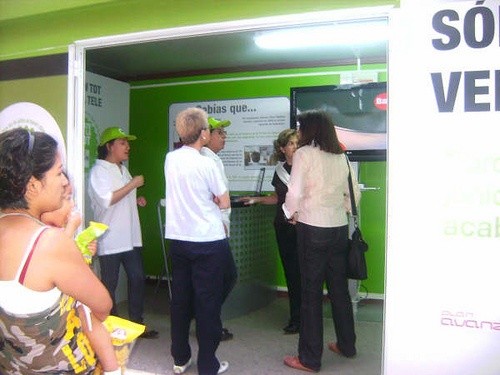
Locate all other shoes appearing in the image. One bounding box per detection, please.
[140,329,158,338]
[282,323,300,335]
[328,341,342,352]
[283,354,317,372]
[216,360,229,374]
[220,328,234,341]
[173,357,192,374]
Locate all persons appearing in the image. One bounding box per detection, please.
[282,110,362,373]
[87,127,159,338]
[200,116,235,342]
[39,170,129,375]
[163,107,230,374]
[0,128,115,375]
[240,129,302,336]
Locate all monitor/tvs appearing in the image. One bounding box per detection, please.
[290,81,387,162]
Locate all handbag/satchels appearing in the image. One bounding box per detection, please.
[345,228,369,279]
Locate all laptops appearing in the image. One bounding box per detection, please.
[232,168,265,199]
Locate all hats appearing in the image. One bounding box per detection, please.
[206,116,231,131]
[99,127,137,147]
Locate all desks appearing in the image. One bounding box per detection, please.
[157,195,267,321]
